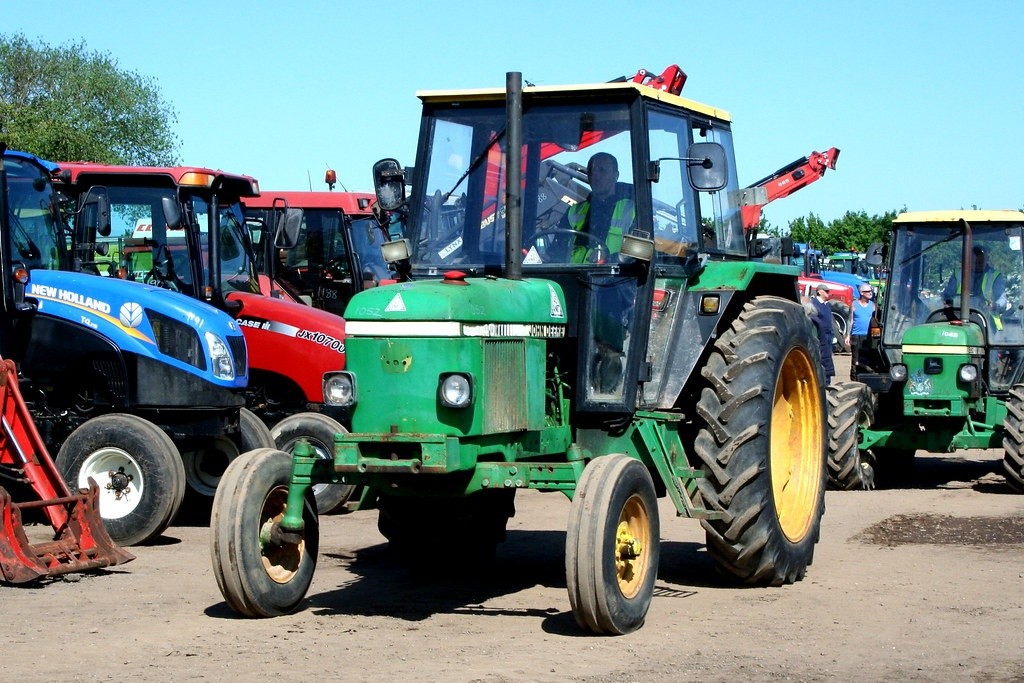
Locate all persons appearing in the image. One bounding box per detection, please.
[844,283,877,381]
[545,152,638,265]
[807,284,836,386]
[942,245,1007,334]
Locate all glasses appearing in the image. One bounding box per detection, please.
[862,290,872,293]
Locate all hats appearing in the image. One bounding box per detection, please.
[815,284,830,293]
[972,245,988,256]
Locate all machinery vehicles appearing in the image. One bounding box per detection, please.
[238,191,398,317]
[750,237,884,353]
[211,62,830,636]
[49,162,359,516]
[828,211,1024,490]
[0,149,278,547]
[738,148,840,251]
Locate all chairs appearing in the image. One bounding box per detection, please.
[615,181,635,199]
[112,252,135,281]
